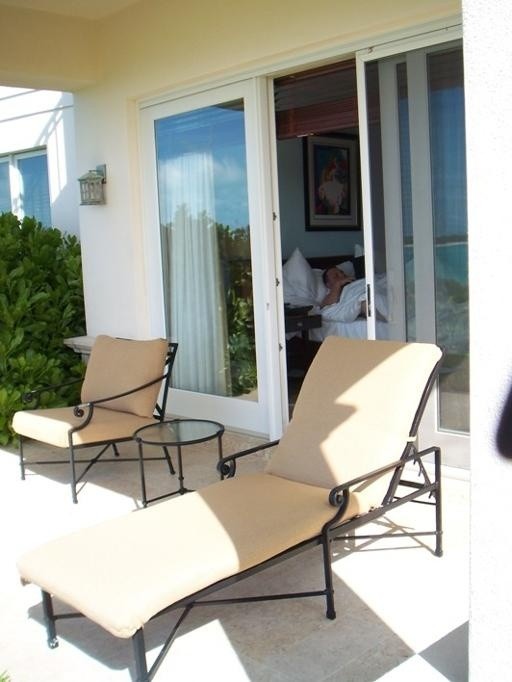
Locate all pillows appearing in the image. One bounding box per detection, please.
[284,247,357,310]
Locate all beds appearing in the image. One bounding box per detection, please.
[284,255,468,357]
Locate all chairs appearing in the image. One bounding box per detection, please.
[12,340,444,682]
[8,337,179,502]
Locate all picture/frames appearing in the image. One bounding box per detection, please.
[302,132,361,232]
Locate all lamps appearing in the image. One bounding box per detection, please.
[77,164,106,206]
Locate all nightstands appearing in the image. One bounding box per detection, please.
[283,302,322,374]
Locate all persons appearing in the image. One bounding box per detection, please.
[320,265,388,324]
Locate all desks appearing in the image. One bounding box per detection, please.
[134,419,225,506]
